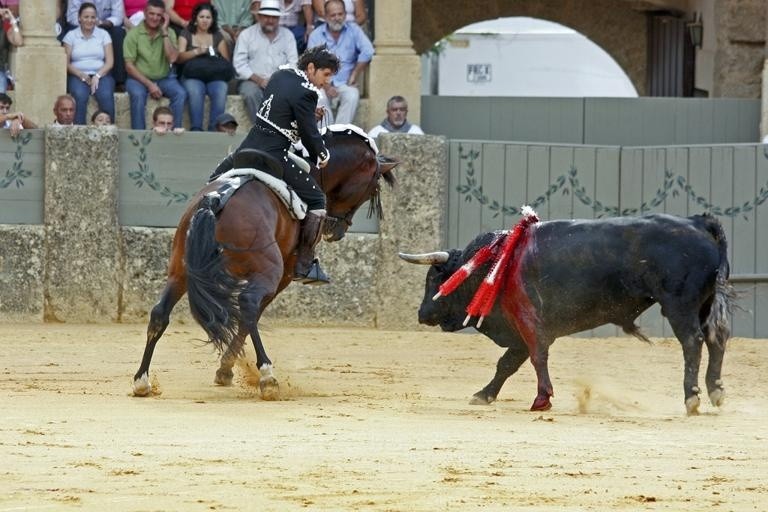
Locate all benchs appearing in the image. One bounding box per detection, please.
[72,91,371,129]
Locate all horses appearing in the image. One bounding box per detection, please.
[132,125,401,399]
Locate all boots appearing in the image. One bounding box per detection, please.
[295,211,330,281]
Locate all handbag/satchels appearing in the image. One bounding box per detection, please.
[182,52,238,83]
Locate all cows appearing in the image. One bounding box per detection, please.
[398,211,734,416]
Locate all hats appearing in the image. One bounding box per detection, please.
[215,113,238,127]
[250,1,288,18]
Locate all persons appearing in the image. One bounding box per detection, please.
[62,2,115,126]
[204,45,340,287]
[309,0,374,123]
[215,113,238,132]
[53,95,76,126]
[234,1,299,123]
[54,0,370,94]
[151,107,184,134]
[91,111,112,125]
[0,93,37,138]
[368,96,428,137]
[0,0,23,92]
[124,0,187,129]
[179,0,228,131]
